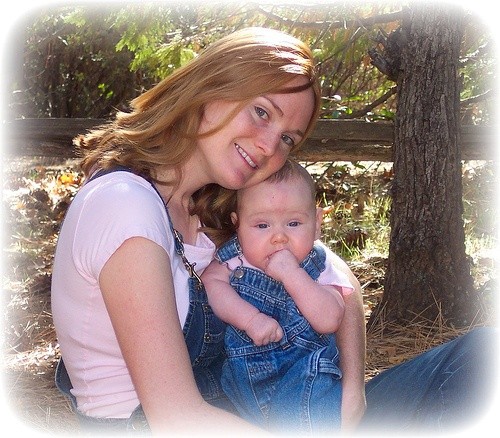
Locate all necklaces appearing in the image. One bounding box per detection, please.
[173,228,219,286]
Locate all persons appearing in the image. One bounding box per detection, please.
[200,156,345,435]
[47,26,497,434]
